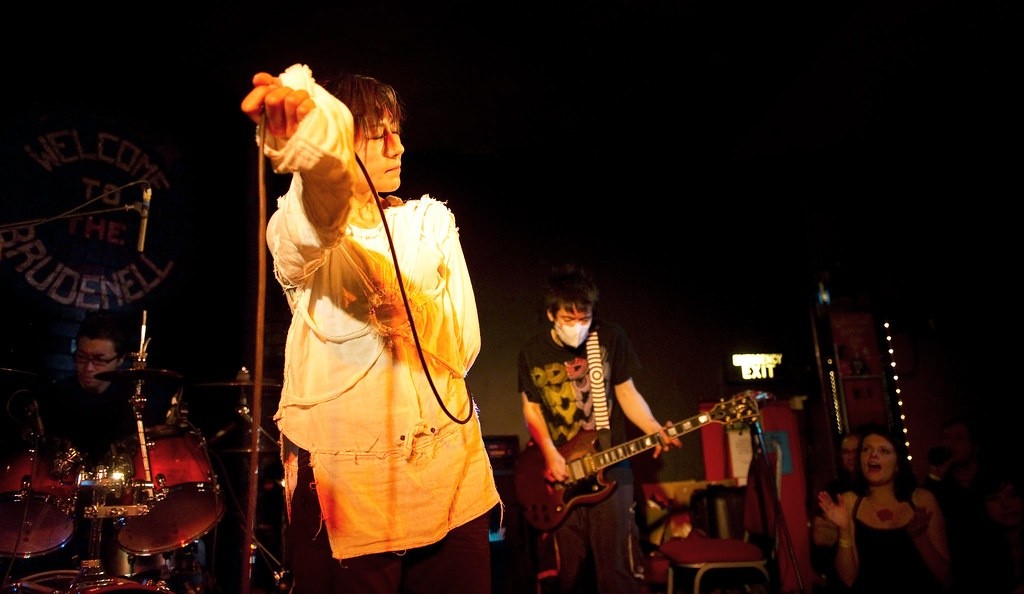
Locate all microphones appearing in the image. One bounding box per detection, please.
[137,184,152,252]
[30,394,45,434]
[165,389,183,425]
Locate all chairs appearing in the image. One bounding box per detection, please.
[660,440,782,594]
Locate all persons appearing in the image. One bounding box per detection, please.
[517,269,683,594]
[47,310,208,594]
[808,418,1024,594]
[241,62,503,594]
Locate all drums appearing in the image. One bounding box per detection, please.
[0,419,227,594]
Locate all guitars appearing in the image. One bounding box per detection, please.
[510,388,762,533]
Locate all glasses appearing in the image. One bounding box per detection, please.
[73,347,120,368]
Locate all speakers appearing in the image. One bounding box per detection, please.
[487,470,540,594]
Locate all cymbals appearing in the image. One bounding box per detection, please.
[92,366,184,391]
[0,366,41,377]
[216,443,279,455]
[190,377,283,388]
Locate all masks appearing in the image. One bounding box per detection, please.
[552,318,593,349]
